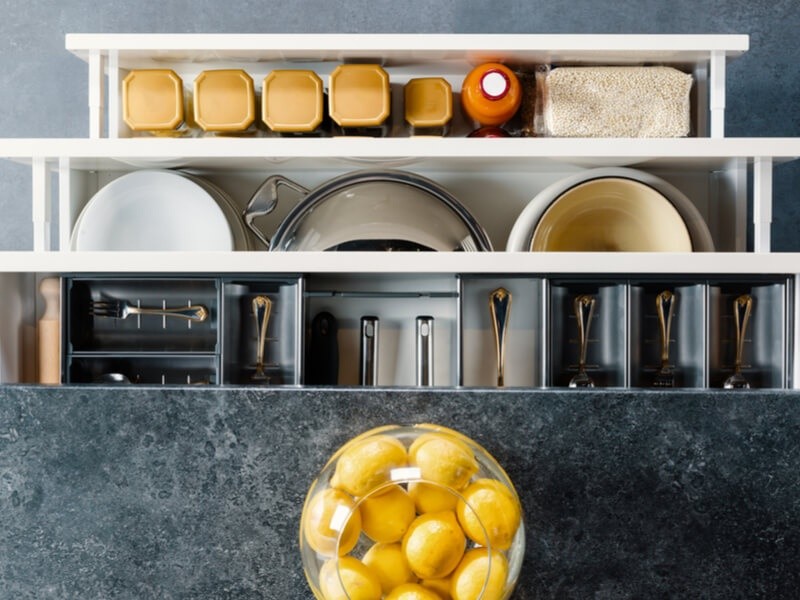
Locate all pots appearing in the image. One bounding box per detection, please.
[242,173,492,251]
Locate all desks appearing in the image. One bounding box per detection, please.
[0,386,800,600]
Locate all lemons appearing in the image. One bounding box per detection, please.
[304,432,520,600]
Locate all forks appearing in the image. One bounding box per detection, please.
[89,300,207,323]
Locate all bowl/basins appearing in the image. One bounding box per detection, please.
[505,167,715,253]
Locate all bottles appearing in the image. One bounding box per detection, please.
[193,68,262,139]
[261,69,333,137]
[328,65,391,138]
[404,78,453,137]
[122,68,201,138]
[463,62,522,126]
[469,127,510,137]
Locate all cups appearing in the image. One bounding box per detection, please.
[298,424,525,600]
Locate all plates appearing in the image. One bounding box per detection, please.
[70,166,253,251]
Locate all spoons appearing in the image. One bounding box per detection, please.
[91,373,210,385]
